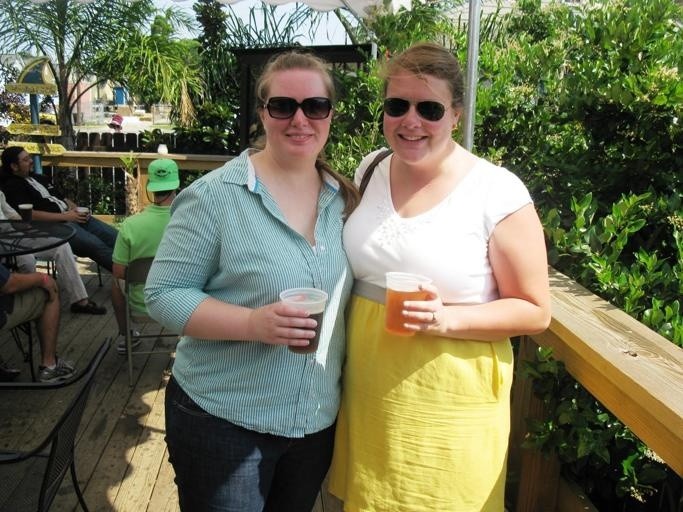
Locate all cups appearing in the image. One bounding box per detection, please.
[74,206,90,227]
[18,203,35,221]
[277,285,330,354]
[377,270,434,340]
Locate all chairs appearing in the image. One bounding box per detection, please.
[123,257,182,387]
[0,173,103,382]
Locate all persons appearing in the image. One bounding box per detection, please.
[139,48,363,511]
[341,42,554,512]
[0,142,120,385]
[109,158,182,357]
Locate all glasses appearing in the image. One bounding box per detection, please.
[264,96,333,121]
[382,96,445,121]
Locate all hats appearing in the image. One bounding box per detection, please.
[147,158,181,193]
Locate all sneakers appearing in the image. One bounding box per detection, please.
[71,300,108,314]
[38,356,77,383]
[117,329,141,355]
[0,363,22,381]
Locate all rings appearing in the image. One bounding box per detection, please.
[431,312,440,326]
